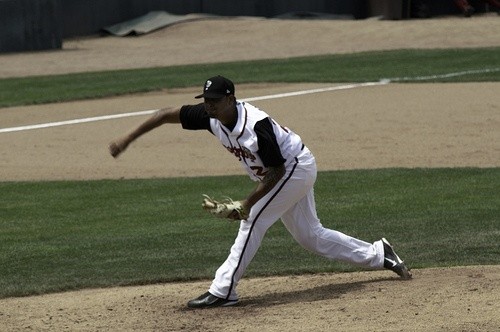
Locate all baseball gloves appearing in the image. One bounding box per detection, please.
[202,192,249,222]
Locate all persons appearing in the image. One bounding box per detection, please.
[110,75,412,308]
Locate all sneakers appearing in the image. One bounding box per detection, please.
[381,237,413,279]
[187,290,238,309]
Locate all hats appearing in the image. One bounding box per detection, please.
[195,75,235,99]
